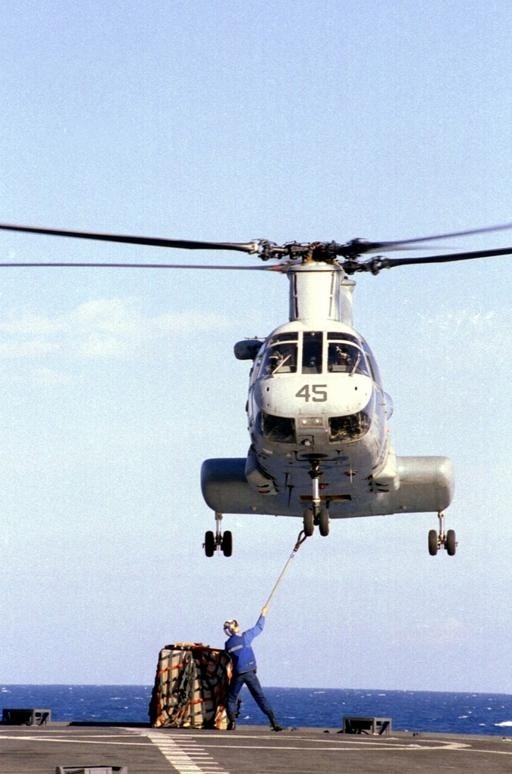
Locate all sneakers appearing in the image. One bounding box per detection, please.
[228,721,235,730]
[274,725,282,731]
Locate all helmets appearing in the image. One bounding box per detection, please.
[223,619,240,635]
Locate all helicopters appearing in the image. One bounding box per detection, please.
[1,220,510,559]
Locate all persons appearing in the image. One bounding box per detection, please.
[222,607,286,732]
